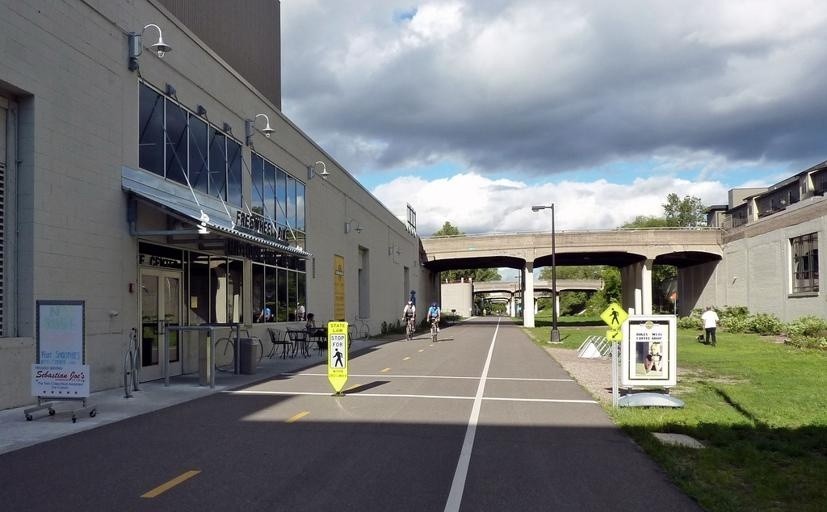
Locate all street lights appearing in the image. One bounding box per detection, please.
[530,204,560,340]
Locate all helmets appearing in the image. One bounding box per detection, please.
[432,303,437,306]
[407,301,412,304]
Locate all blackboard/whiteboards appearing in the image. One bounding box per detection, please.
[31,299,91,399]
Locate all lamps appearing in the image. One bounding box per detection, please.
[245,113,276,138]
[127,23,173,61]
[308,161,331,181]
[345,218,425,267]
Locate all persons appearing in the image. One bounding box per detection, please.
[294,301,305,321]
[644,343,661,373]
[700,305,719,347]
[305,312,324,337]
[400,300,415,336]
[426,302,440,338]
[258,305,272,321]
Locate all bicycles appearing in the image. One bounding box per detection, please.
[403,316,416,341]
[256,314,275,323]
[213,322,263,372]
[296,311,303,321]
[428,318,438,342]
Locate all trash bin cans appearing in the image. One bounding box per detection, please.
[233,338,258,375]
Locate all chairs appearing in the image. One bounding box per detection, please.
[266,325,328,360]
